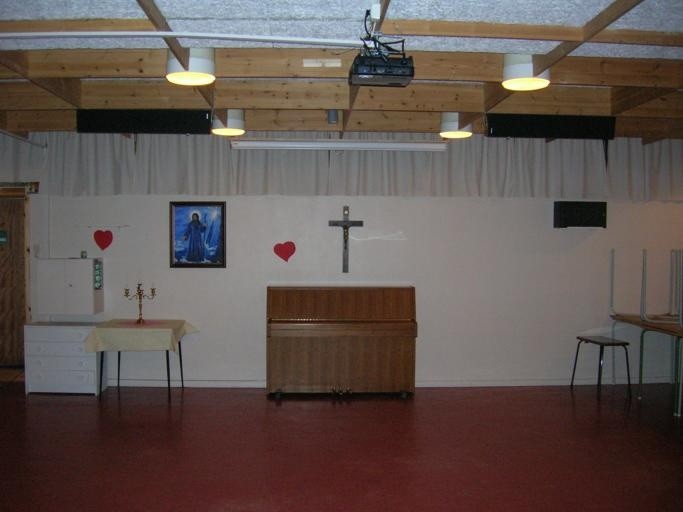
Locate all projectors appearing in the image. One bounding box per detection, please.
[349,54,414,87]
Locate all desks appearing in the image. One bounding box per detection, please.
[603,318,682,423]
[608,247,682,335]
[81,320,196,407]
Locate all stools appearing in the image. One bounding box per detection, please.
[567,332,637,403]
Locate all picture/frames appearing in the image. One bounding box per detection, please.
[168,199,228,270]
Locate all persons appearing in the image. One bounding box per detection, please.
[182,212,208,263]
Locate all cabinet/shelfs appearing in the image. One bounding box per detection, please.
[30,257,105,316]
[23,320,109,396]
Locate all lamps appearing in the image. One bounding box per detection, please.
[327,108,338,125]
[209,107,247,138]
[164,47,216,88]
[497,52,553,92]
[438,110,474,142]
[227,137,450,154]
[118,279,159,324]
